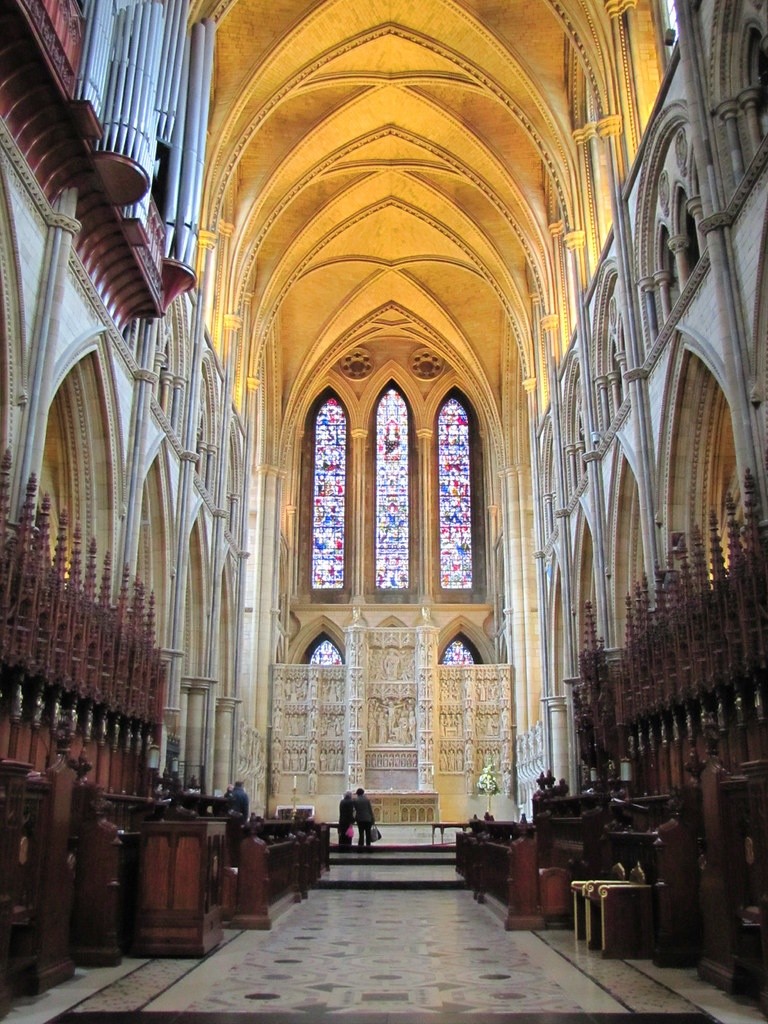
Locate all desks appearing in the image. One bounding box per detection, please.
[352,790,440,825]
[430,822,471,847]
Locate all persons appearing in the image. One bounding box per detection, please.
[224,780,248,823]
[337,789,374,846]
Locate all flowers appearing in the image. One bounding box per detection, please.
[478,764,500,796]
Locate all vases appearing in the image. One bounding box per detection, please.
[485,789,494,814]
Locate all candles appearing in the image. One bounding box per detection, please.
[293,775,297,788]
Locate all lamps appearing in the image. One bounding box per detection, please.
[619,758,632,781]
[171,756,178,772]
[147,745,160,771]
[590,767,597,782]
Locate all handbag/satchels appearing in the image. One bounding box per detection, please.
[377,831,382,840]
[369,823,378,842]
[345,824,354,838]
